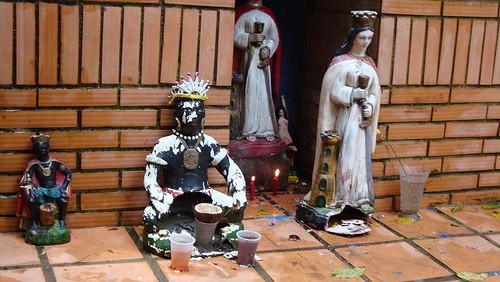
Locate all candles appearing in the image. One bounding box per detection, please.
[249,175,256,201]
[272,169,281,196]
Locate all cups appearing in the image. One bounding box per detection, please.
[169,234,196,271]
[195,218,219,247]
[399,166,430,214]
[236,230,261,264]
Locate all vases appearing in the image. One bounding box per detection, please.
[399,164,431,220]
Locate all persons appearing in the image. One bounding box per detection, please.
[140,70,249,227]
[234,0,281,142]
[300,9,384,215]
[16,131,74,232]
[277,109,293,146]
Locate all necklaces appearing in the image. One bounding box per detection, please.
[38,162,52,176]
[171,128,204,141]
[176,135,204,170]
